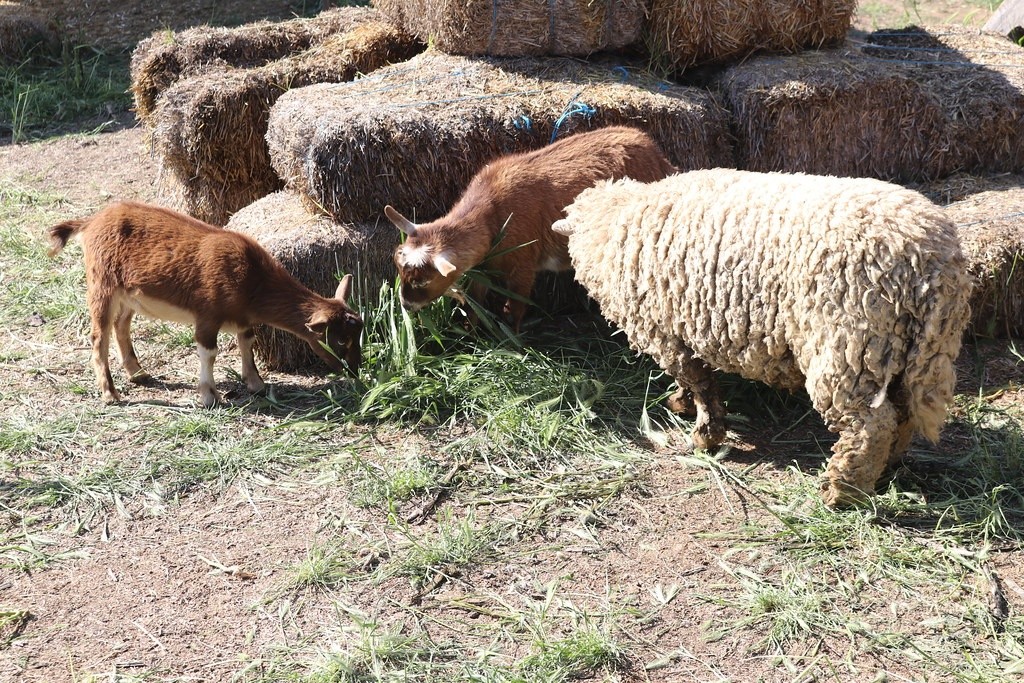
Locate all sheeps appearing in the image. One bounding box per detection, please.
[383,126,679,334]
[46,201,366,408]
[551,164,977,508]
